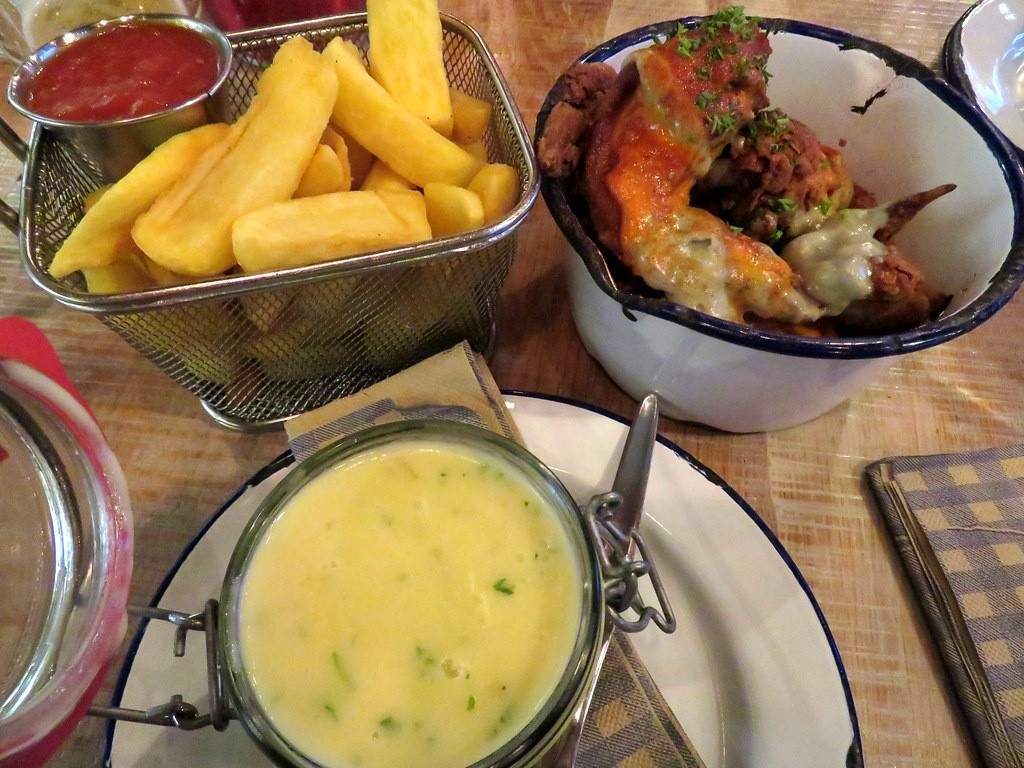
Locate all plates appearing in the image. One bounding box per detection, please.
[102,391,867,768]
[943,0,1023,170]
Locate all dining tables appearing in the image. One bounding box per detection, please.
[2,0,1023,768]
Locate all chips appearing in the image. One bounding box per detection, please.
[48,2,523,383]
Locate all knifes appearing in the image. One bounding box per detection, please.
[520,393,660,765]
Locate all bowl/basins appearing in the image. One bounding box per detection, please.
[89,419,676,767]
[6,13,234,182]
[535,15,1023,434]
